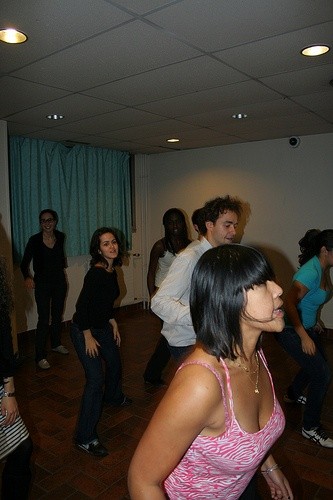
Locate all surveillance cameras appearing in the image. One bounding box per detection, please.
[288,136,300,148]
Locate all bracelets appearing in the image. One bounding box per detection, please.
[260,464,279,473]
[4,392,16,398]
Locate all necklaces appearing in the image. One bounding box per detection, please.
[242,352,260,394]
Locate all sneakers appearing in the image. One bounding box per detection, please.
[74,438,109,458]
[282,393,308,405]
[104,398,130,408]
[300,425,333,448]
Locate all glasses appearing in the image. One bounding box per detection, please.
[40,218,54,223]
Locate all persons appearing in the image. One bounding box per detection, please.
[127,245,294,499]
[282,228,333,447]
[71,228,127,459]
[144,208,189,392]
[150,195,242,365]
[19,210,70,370]
[186,208,208,251]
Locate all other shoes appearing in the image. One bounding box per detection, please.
[143,377,166,387]
[37,358,50,369]
[52,345,71,355]
